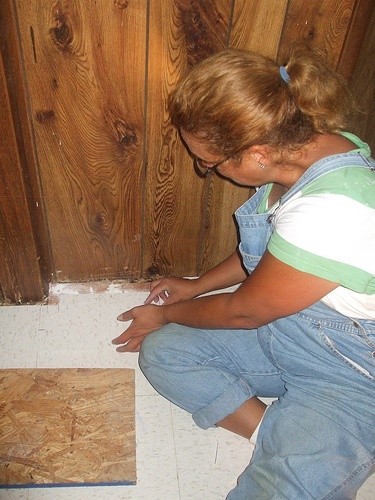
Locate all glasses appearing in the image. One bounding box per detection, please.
[197,138,264,176]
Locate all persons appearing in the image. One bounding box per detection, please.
[110,45,375,500]
